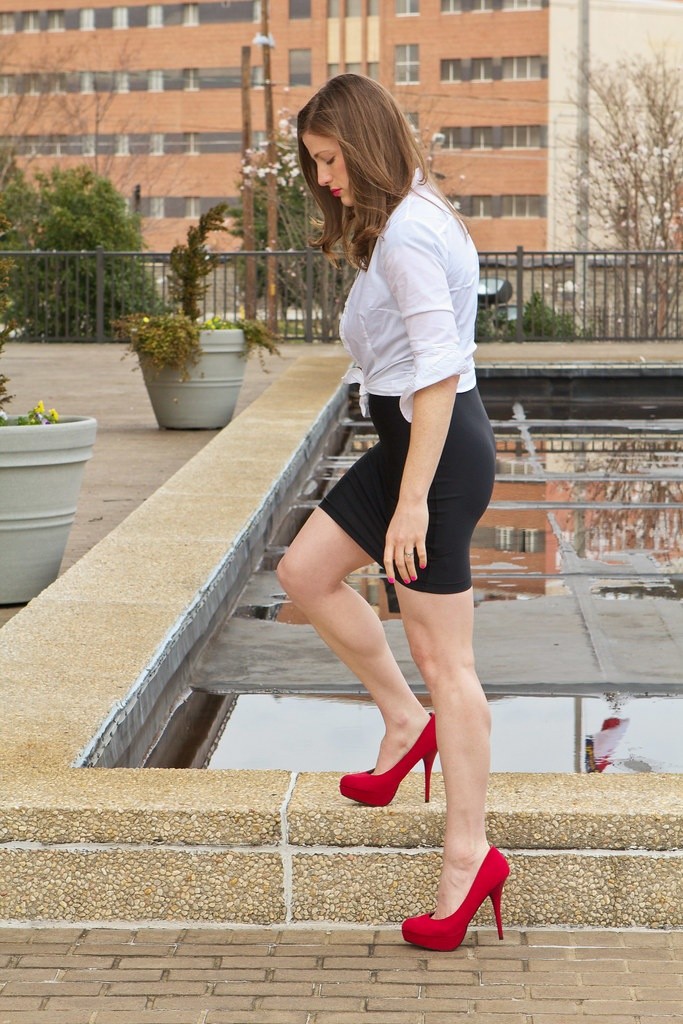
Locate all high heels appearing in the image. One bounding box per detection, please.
[338,712,436,806]
[401,845,510,951]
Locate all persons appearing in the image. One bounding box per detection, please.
[273,73,510,953]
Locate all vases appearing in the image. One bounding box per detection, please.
[135,328,245,429]
[1,416,99,605]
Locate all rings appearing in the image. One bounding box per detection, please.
[404,552,413,556]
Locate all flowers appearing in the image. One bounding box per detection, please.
[108,201,284,382]
[1,258,60,425]
